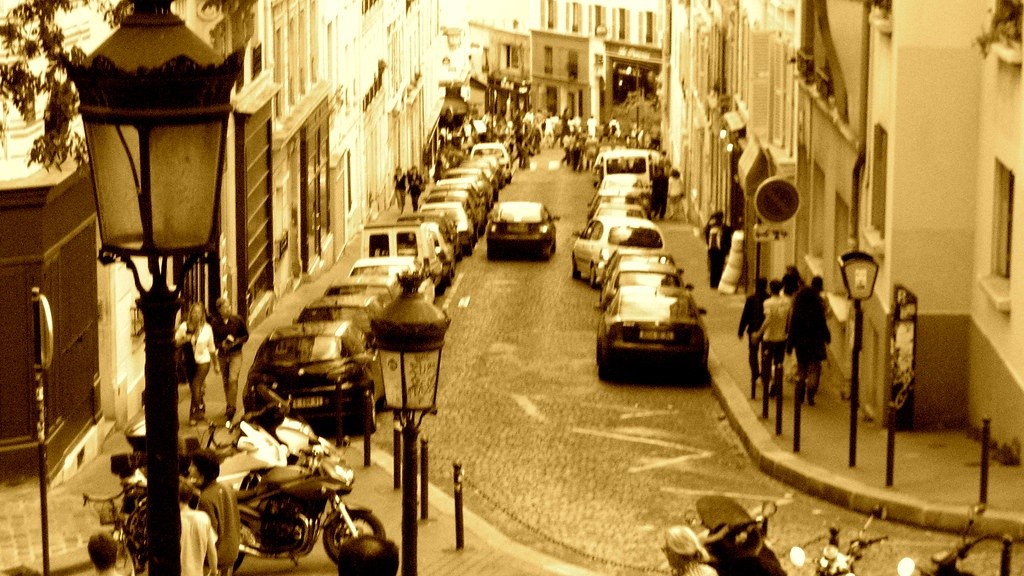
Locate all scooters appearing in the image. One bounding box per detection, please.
[664,490,1004,576]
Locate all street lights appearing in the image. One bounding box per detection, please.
[371,270,452,575]
[64,1,248,576]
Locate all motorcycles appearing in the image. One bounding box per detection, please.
[190,382,384,575]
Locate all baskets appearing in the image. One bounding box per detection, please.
[93,495,125,525]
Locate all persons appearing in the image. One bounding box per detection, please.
[179,476,219,576]
[392,167,407,216]
[508,105,562,170]
[661,526,719,576]
[461,103,508,149]
[211,298,249,421]
[563,108,653,153]
[649,151,684,220]
[737,266,831,406]
[707,211,731,291]
[561,130,597,173]
[175,301,220,426]
[407,166,422,213]
[189,447,240,576]
[88,532,123,576]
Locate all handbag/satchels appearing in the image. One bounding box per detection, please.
[175,346,187,384]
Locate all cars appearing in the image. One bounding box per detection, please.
[293,141,514,345]
[241,320,388,435]
[597,247,696,311]
[569,214,666,290]
[485,201,562,264]
[586,149,661,219]
[596,285,711,387]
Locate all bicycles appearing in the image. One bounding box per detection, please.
[83,482,127,573]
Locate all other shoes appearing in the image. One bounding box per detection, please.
[226,409,235,422]
[189,419,197,426]
